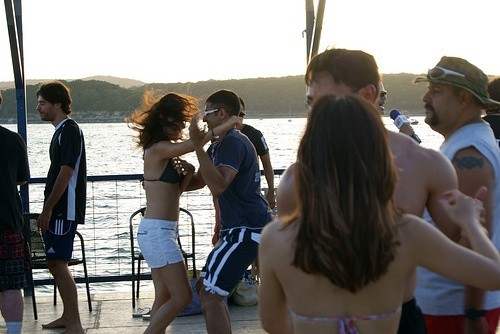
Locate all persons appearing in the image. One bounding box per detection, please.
[35,82,87,334]
[0,93,31,334]
[141,90,274,334]
[255,47,500,333]
[126,90,243,334]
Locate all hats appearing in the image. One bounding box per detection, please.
[414,56,500,106]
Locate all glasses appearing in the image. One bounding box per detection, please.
[239,112,246,117]
[426,67,465,80]
[205,109,220,116]
[378,92,387,98]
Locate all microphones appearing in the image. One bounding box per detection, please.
[390,110,421,143]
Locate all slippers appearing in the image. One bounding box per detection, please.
[141,314,151,320]
[132,306,152,316]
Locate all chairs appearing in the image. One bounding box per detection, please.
[20,212,92,321]
[129,207,197,310]
